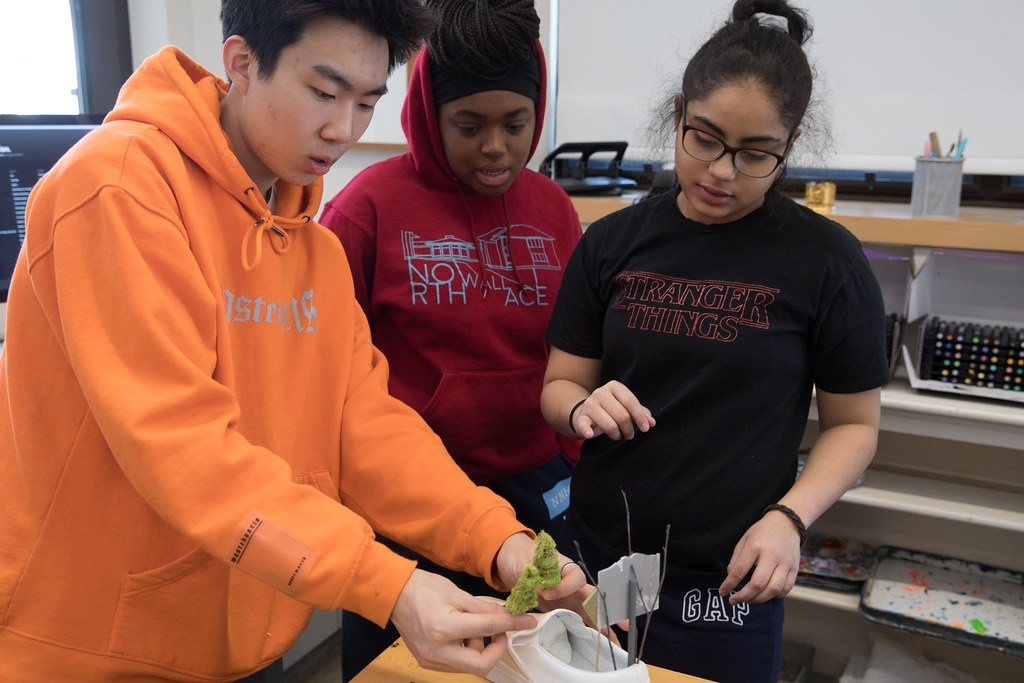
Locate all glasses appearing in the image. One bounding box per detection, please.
[681,96,796,178]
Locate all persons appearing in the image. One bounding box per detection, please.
[540,0,886,683]
[0,0,632,683]
[320,0,584,683]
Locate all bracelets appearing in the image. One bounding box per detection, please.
[569,399,590,435]
[764,505,806,545]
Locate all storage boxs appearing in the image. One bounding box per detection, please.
[911,155,966,223]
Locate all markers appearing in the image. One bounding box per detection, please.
[920,316,1024,392]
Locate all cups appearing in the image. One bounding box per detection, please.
[910,155,966,220]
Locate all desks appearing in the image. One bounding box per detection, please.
[349,637,713,683]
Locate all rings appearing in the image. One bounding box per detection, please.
[560,562,580,580]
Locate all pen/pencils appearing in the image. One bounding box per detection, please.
[924,127,967,159]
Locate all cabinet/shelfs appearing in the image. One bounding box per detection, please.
[569,196,1024,617]
